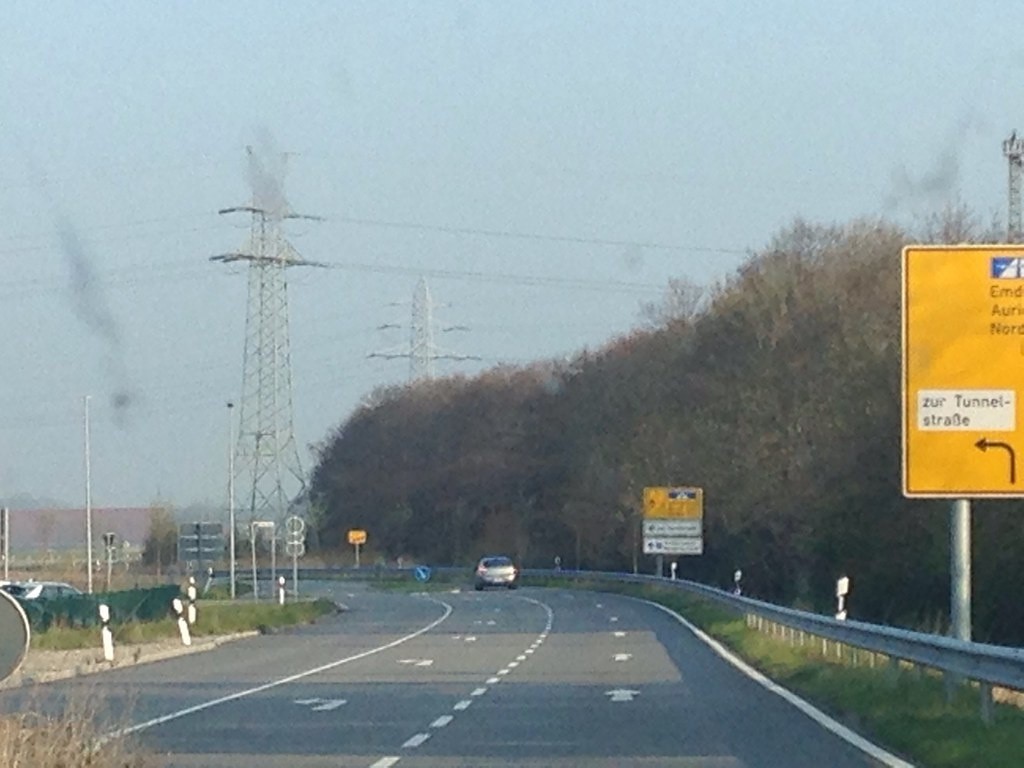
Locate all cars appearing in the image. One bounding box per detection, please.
[1,580,81,600]
[474,556,517,592]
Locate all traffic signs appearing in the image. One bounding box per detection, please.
[899,244,1024,500]
[640,485,703,555]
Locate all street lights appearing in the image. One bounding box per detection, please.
[225,400,236,600]
[83,395,95,596]
